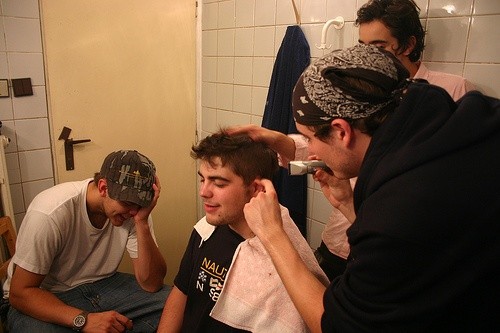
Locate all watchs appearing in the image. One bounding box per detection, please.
[73,310,88,333]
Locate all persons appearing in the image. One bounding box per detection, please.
[156,125,330,332]
[3,150,175,333]
[244,42,500,333]
[218,0,485,283]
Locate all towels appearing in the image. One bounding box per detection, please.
[193,203,330,333]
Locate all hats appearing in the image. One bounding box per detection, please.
[100,150,156,208]
